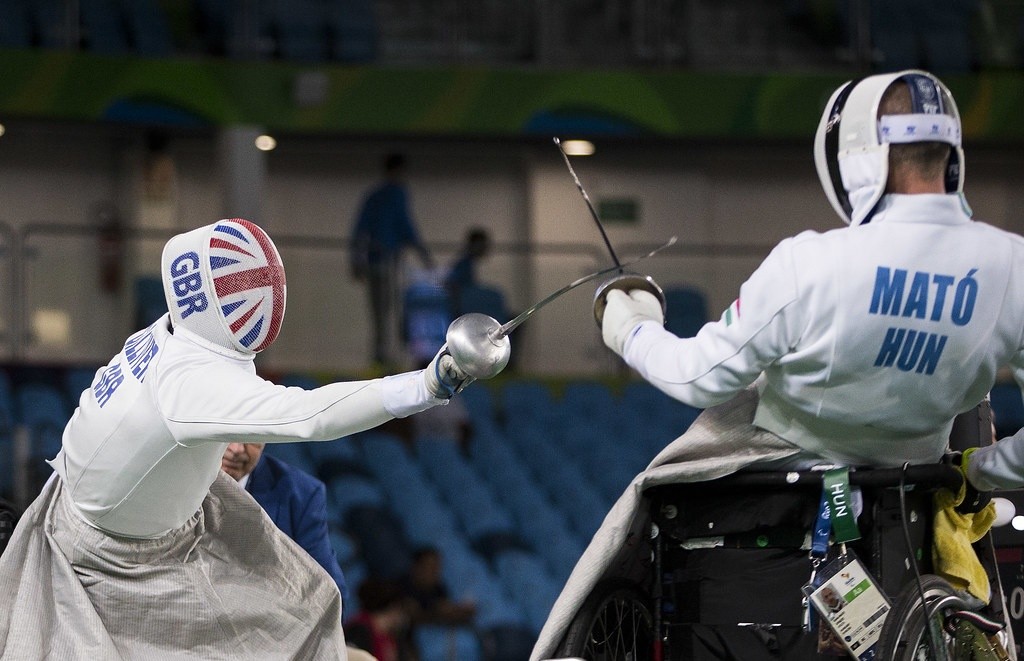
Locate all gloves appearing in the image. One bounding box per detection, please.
[601,286,664,357]
[380,343,475,420]
[952,448,992,516]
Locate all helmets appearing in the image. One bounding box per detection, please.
[162,216,289,356]
[815,69,974,228]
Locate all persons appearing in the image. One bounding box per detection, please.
[358,543,478,661]
[0,218,470,661]
[217,443,378,661]
[602,70,1024,516]
[344,158,512,376]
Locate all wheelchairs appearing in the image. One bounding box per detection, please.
[545,463,1013,661]
